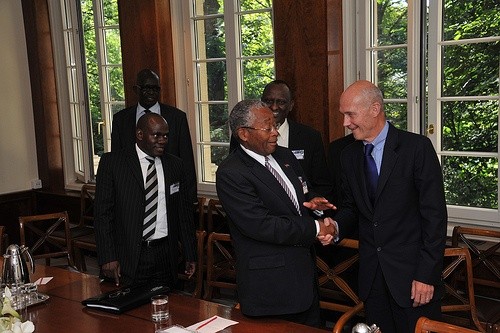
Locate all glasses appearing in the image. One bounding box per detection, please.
[109,288,130,297]
[138,85,160,95]
[240,123,281,133]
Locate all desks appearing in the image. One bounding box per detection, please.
[0,283,167,333]
[0,253,335,333]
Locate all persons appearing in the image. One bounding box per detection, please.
[229,81,326,187]
[316,80,448,333]
[111,71,199,228]
[94,113,198,293]
[216,99,337,330]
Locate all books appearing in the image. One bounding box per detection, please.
[81,286,170,314]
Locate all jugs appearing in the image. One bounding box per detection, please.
[2,244,35,288]
[352,323,371,333]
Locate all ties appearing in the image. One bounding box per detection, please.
[265,156,302,218]
[143,109,151,114]
[363,143,379,211]
[142,155,158,241]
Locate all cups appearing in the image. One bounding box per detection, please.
[155,315,172,333]
[11,283,40,319]
[151,294,169,322]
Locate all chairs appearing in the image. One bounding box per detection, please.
[0,178,500,333]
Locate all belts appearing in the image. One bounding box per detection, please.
[144,240,166,245]
